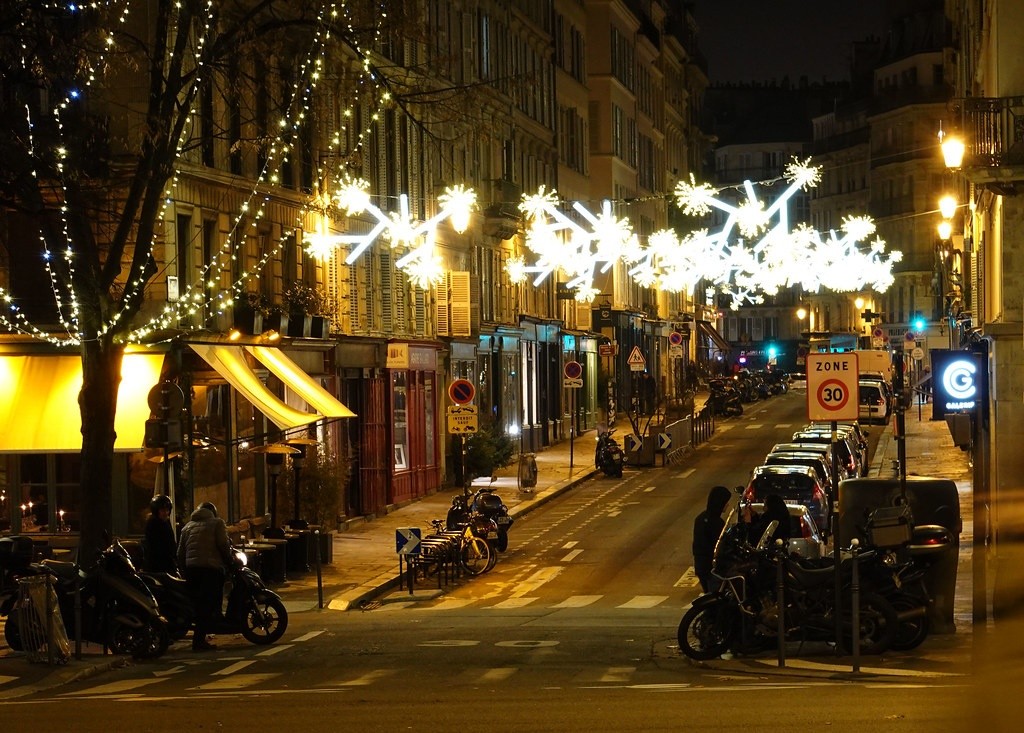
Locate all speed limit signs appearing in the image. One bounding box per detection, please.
[807,354,859,421]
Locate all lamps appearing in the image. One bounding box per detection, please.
[941,134,965,173]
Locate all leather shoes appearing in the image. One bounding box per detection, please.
[192,640,217,650]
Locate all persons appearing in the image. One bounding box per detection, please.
[692,485,733,593]
[919,364,931,404]
[643,373,656,415]
[144,493,179,571]
[176,500,230,652]
[749,493,794,537]
[686,360,698,395]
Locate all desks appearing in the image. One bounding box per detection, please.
[52,548,71,561]
[234,525,325,569]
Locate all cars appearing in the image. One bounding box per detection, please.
[770,443,831,467]
[858,382,887,425]
[793,421,869,478]
[860,379,892,415]
[765,452,832,499]
[714,502,826,571]
[734,465,829,544]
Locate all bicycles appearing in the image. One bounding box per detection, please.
[431,519,496,572]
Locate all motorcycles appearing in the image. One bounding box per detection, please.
[679,487,958,659]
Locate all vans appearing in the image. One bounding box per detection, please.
[786,373,806,389]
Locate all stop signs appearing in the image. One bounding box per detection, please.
[670,332,683,346]
[449,380,474,405]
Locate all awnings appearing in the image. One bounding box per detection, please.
[187,344,326,432]
[243,346,358,419]
[0,355,165,456]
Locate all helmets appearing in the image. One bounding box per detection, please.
[149,494,172,513]
[196,501,218,518]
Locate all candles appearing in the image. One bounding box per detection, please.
[59,509,64,520]
[28,501,34,514]
[20,504,27,518]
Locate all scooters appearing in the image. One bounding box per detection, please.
[701,389,743,417]
[105,541,286,653]
[0,535,168,660]
[729,367,789,403]
[447,487,513,552]
[595,428,624,478]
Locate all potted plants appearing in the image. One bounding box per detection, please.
[235,280,345,338]
[454,418,514,477]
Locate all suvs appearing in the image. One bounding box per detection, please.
[859,371,884,380]
